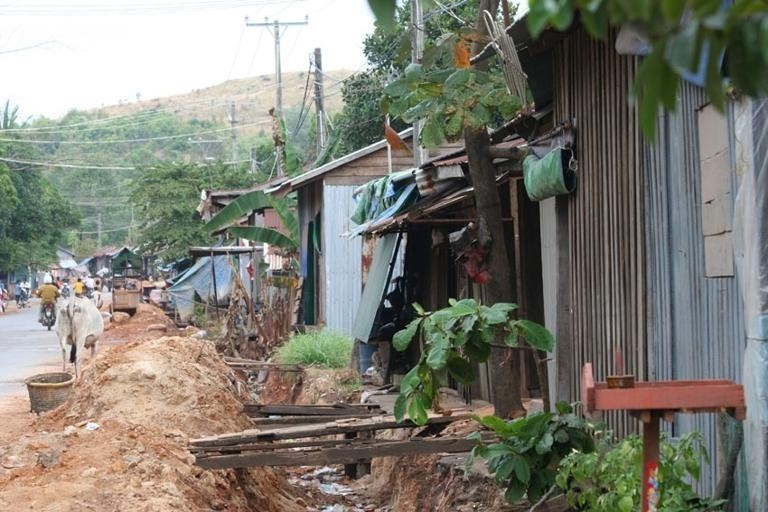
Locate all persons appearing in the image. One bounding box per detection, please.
[0,271,103,324]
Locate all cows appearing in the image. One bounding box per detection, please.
[54,289,105,377]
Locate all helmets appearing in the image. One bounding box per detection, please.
[43,275,52,283]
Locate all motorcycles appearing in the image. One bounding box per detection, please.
[36,289,55,330]
[62,284,70,299]
